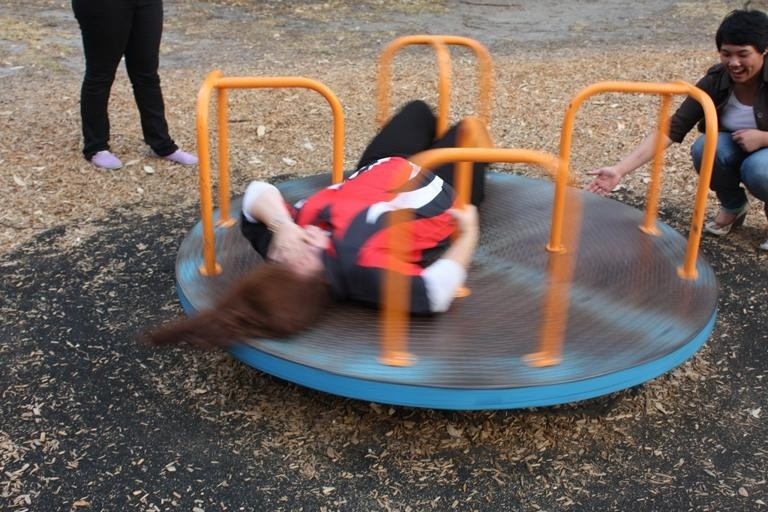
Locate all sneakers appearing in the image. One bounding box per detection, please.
[151,147,198,167]
[92,152,124,170]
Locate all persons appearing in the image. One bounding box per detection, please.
[584,5,768,251]
[69,1,198,170]
[150,100,494,351]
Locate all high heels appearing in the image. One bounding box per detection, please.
[704,206,747,236]
